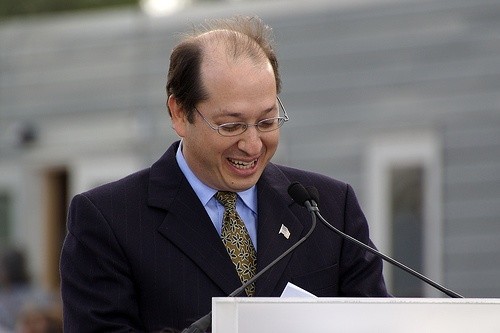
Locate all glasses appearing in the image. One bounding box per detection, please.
[192,96,289,137]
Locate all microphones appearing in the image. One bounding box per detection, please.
[305,183,464,298]
[181,181,317,333]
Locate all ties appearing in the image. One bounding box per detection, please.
[214,191,256,296]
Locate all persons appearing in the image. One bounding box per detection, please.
[59,15,395,333]
